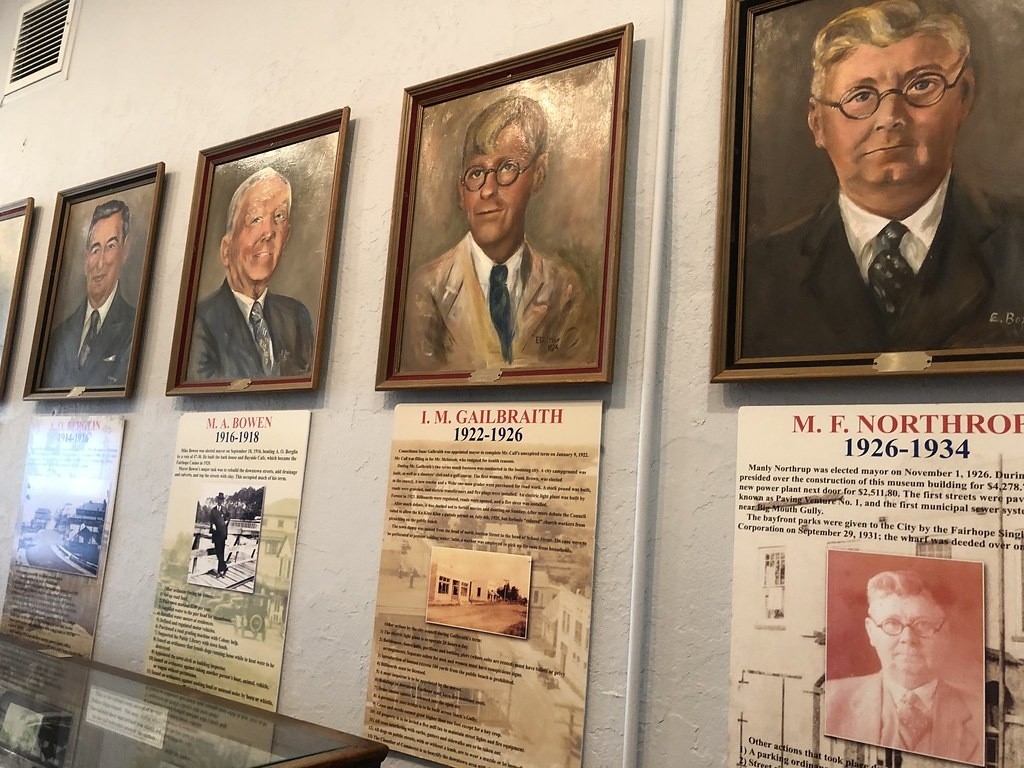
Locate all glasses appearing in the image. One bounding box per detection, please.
[868,616,945,638]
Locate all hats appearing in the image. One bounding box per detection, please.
[216,493,225,499]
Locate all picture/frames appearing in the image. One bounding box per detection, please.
[0,196,34,403]
[707,1,1024,386]
[164,106,351,397]
[21,161,166,399]
[373,22,635,392]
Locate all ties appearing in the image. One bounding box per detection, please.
[898,693,930,749]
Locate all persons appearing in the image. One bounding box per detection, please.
[209,492,231,578]
[826,571,984,764]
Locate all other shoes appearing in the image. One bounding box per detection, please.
[216,572,221,578]
[223,568,228,575]
